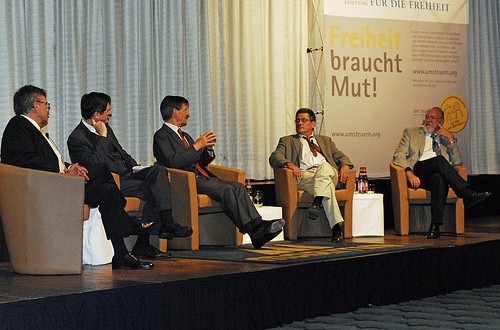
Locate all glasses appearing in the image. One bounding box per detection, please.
[294,119,315,123]
[425,114,443,119]
[36,100,50,109]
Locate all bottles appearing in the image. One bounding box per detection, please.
[254,191,263,206]
[353,166,368,194]
[246,178,254,204]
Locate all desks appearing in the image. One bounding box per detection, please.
[83,205,115,266]
[352,191,385,237]
[243,205,284,245]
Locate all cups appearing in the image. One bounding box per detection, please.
[367,184,376,194]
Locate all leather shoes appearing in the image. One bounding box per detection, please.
[159,223,193,239]
[427,225,441,239]
[331,231,343,242]
[131,244,172,260]
[464,191,491,209]
[112,252,154,269]
[122,216,159,237]
[308,203,320,220]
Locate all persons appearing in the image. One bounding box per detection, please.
[269,107,354,243]
[153,95,286,249]
[392,107,491,239]
[67,92,193,260]
[1,84,155,270]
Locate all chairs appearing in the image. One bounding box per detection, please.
[0,163,90,277]
[124,165,171,253]
[164,163,246,251]
[274,167,356,241]
[390,159,467,236]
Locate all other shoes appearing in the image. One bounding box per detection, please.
[250,218,286,249]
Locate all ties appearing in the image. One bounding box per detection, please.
[430,134,441,155]
[178,128,209,177]
[303,136,325,157]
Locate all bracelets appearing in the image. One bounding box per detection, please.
[449,138,457,144]
[207,146,215,151]
